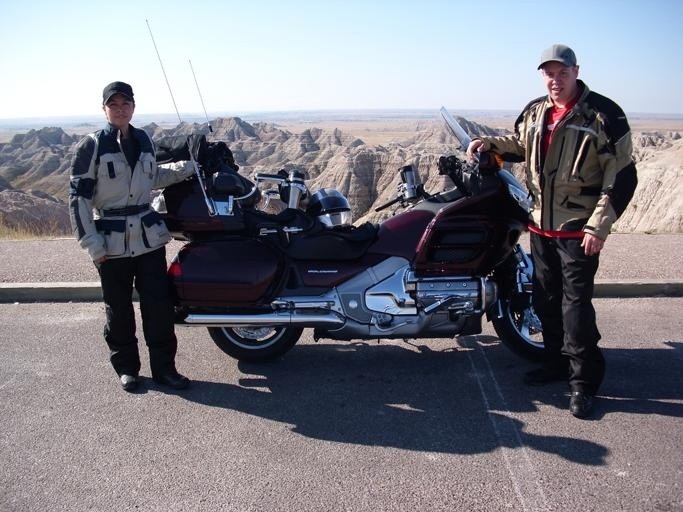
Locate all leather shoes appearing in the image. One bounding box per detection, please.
[569,391,594,417]
[526,369,554,382]
[152,372,189,390]
[120,374,137,390]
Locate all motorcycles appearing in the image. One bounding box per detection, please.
[145,106,546,363]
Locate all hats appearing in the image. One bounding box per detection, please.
[536,44,576,70]
[102,81,134,105]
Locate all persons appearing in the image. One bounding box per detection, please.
[68,80,200,391]
[465,43,638,418]
